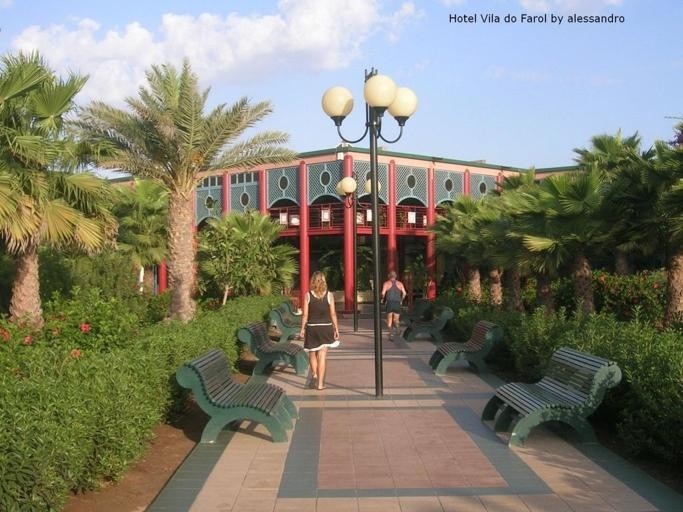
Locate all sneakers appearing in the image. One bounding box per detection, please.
[388,329,400,340]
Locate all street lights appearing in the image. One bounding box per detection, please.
[335,172,382,332]
[322,67,418,399]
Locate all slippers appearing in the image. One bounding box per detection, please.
[309,376,328,390]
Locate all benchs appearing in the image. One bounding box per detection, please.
[480,346,622,449]
[269,299,302,342]
[235,320,308,375]
[399,297,456,344]
[177,346,299,445]
[428,319,503,377]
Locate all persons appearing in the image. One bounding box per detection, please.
[379,270,406,342]
[298,271,340,390]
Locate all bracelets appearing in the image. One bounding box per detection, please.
[333,328,337,331]
